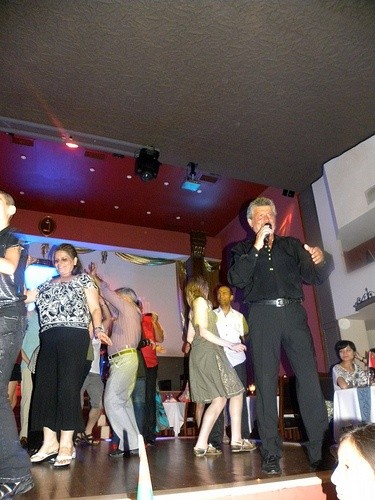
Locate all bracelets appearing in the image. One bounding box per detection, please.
[227,343,237,350]
[100,302,106,306]
[93,326,103,339]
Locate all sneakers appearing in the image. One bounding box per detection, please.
[310,459,337,471]
[260,454,282,474]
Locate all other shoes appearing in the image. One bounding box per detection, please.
[230,438,256,452]
[109,448,139,458]
[0,475,35,500]
[20,437,27,449]
[193,443,222,457]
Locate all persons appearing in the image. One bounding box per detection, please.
[228,197,337,473]
[74,290,113,447]
[0,189,36,500]
[184,274,258,456]
[22,243,113,467]
[129,299,170,454]
[6,289,46,457]
[208,284,250,452]
[85,260,143,458]
[332,340,369,390]
[330,421,375,500]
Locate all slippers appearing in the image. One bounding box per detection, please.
[30,450,58,462]
[53,448,77,467]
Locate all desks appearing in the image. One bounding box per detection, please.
[334,387,375,447]
[113,356,191,447]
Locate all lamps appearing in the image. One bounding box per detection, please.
[133,149,159,182]
[180,162,200,192]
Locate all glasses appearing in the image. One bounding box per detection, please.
[54,258,71,264]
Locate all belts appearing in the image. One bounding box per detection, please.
[250,297,301,308]
[109,349,137,359]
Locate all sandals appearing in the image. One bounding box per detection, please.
[73,436,82,447]
[81,431,100,446]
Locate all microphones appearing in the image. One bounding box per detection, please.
[263,222,272,242]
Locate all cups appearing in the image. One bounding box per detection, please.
[365,369,374,380]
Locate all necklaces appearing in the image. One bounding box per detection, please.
[60,276,67,281]
